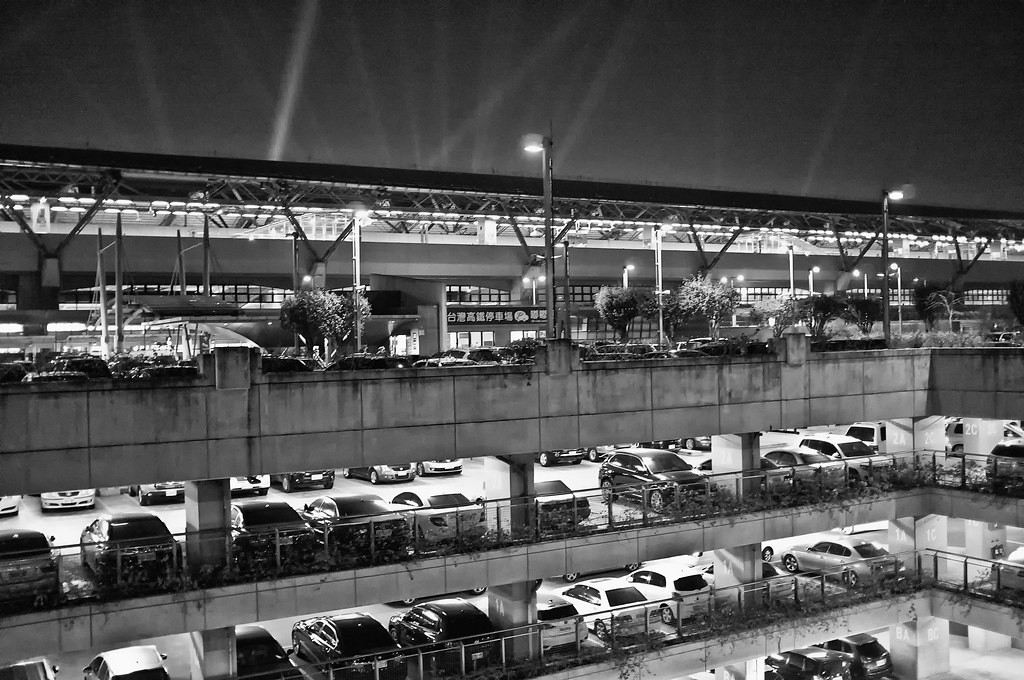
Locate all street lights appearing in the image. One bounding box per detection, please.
[882,189,905,345]
[521,136,559,342]
[622,262,637,341]
[807,265,819,331]
[890,260,904,340]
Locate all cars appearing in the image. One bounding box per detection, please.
[0,413,1023,618]
[8,335,820,379]
[0,534,1024,680]
